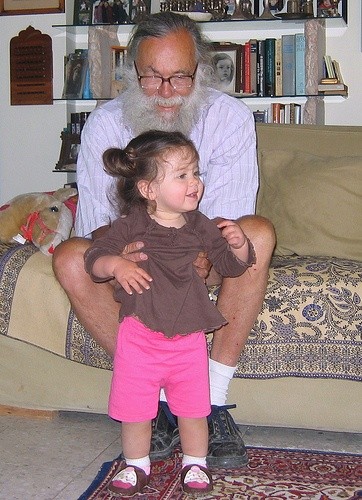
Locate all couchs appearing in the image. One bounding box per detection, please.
[0,120,362,435]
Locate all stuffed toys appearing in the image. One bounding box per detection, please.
[0,188,79,257]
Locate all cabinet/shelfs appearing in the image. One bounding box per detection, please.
[52,0,349,173]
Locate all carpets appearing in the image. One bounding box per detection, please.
[78,442,362,499]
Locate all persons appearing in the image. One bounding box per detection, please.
[52,12,276,469]
[320,0,336,7]
[66,63,81,96]
[268,0,278,9]
[213,55,231,85]
[83,129,255,495]
[94,0,146,23]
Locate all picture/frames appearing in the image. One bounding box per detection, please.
[0,0,65,16]
[204,44,243,94]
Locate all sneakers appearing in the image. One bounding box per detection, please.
[107,463,152,497]
[148,400,181,462]
[205,404,249,468]
[181,464,213,495]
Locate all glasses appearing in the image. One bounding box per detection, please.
[134,60,198,89]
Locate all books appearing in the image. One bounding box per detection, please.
[238,33,306,96]
[253,102,306,125]
[70,112,91,135]
[318,56,347,95]
